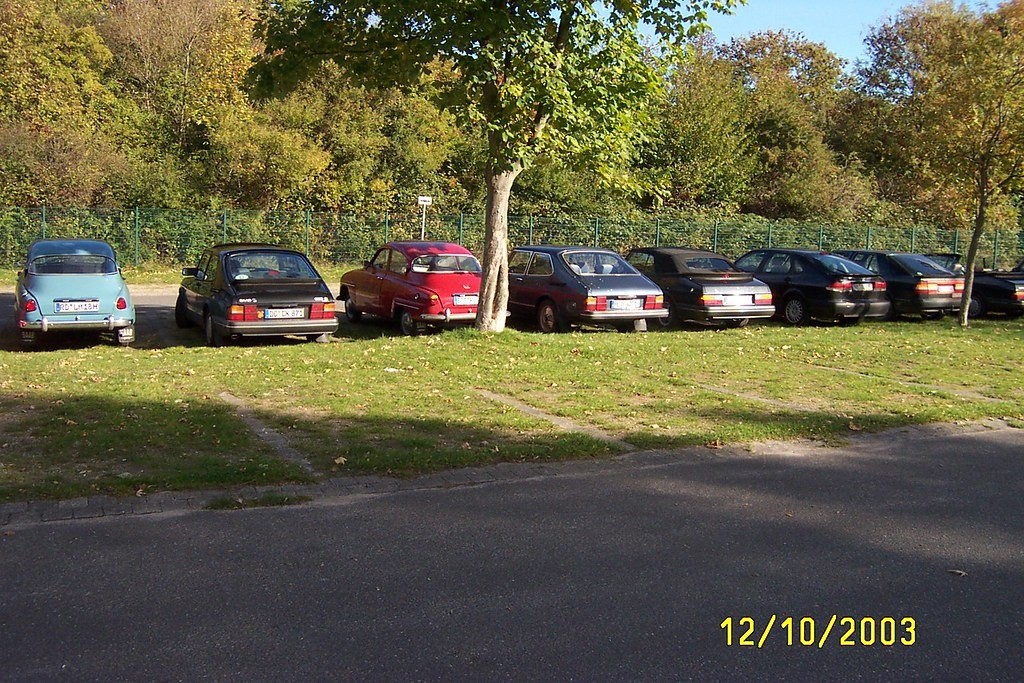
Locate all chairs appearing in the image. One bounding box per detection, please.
[594,264,617,274]
[231,259,240,267]
[952,264,962,272]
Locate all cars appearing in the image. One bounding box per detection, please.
[731,248,891,328]
[14,238,136,348]
[175,244,340,348]
[508,245,669,335]
[922,251,1024,320]
[623,247,776,330]
[832,249,968,321]
[336,241,511,336]
